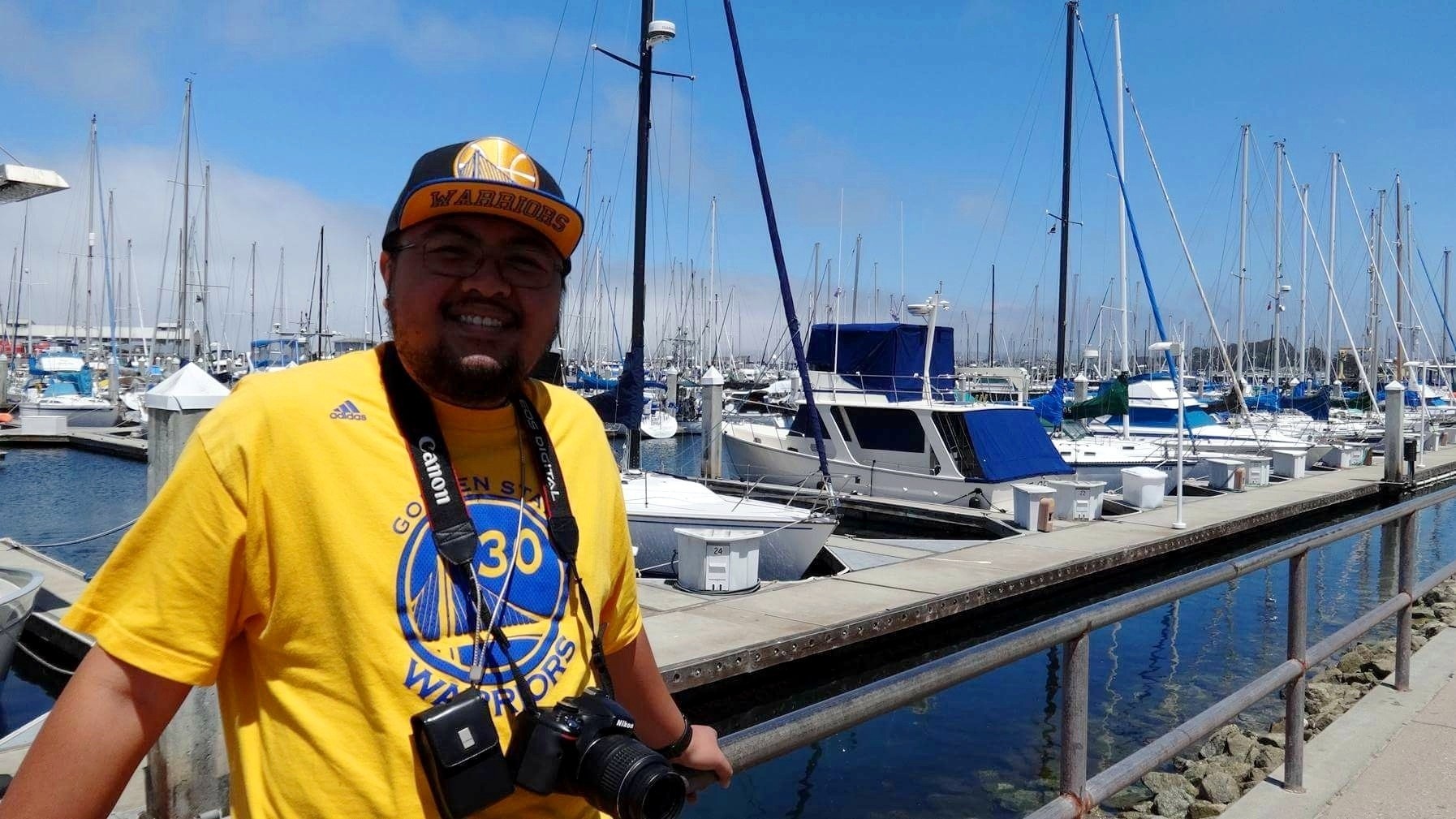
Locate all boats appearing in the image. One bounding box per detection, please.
[0,538,109,664]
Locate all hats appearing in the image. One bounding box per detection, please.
[382,138,584,266]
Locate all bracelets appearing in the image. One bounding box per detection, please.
[646,712,693,761]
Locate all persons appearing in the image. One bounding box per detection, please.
[0,132,734,819]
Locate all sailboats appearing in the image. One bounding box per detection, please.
[0,1,1454,594]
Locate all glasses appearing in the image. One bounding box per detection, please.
[392,231,564,290]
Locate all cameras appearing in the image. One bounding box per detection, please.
[507,688,689,819]
